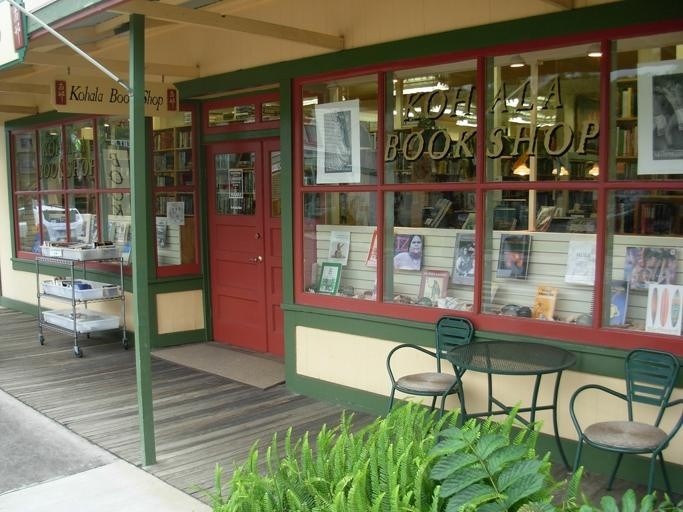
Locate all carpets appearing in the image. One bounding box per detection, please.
[150,345,285,391]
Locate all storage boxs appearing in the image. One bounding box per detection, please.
[40,307,120,334]
[41,277,121,299]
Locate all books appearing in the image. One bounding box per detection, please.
[78,215,167,247]
[375,134,522,230]
[523,128,598,233]
[319,231,377,294]
[395,233,475,307]
[497,234,596,321]
[616,89,682,237]
[608,245,683,335]
[153,130,253,214]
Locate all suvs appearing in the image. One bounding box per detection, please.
[17,206,83,247]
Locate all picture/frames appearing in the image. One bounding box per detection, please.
[315,262,342,295]
[417,269,449,307]
[636,60,683,174]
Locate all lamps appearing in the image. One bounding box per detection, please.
[509,54,525,68]
[586,44,602,57]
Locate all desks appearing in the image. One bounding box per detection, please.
[447,340,576,470]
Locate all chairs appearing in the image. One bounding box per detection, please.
[385,315,473,436]
[569,349,683,505]
